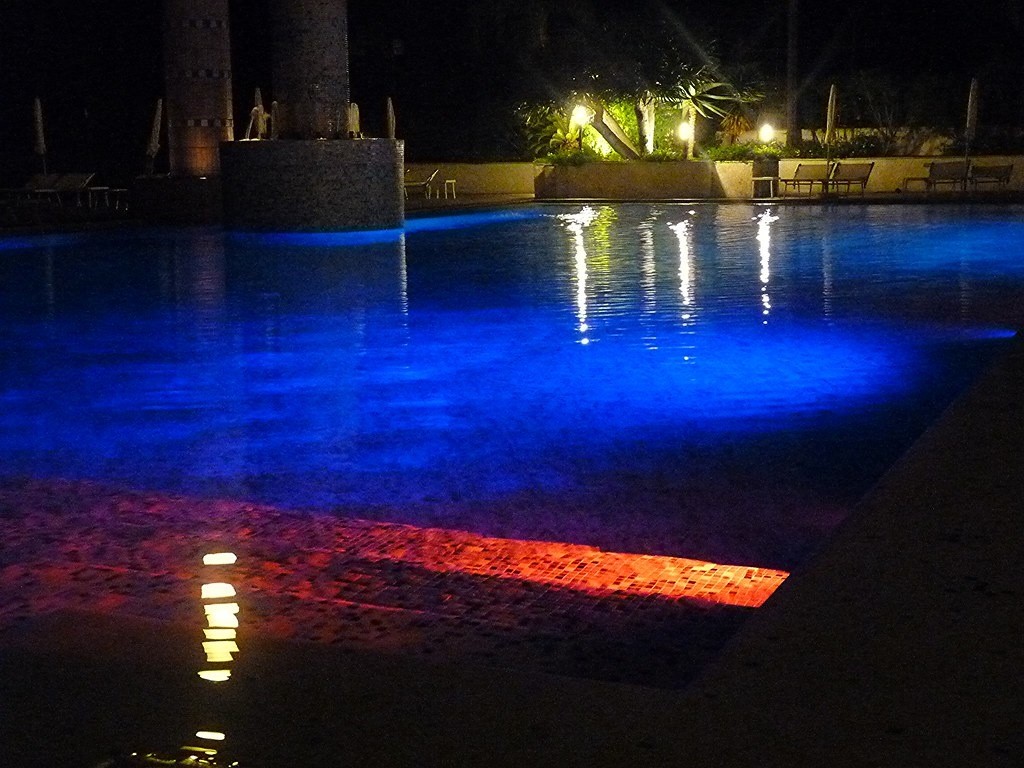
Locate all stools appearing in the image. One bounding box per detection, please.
[109,189,129,212]
[86,187,109,208]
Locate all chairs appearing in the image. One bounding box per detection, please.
[34,173,95,207]
[817,162,875,198]
[779,163,836,199]
[403,169,440,200]
[900,159,971,192]
[964,164,1014,191]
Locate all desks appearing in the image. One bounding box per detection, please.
[435,179,457,199]
[750,177,782,198]
